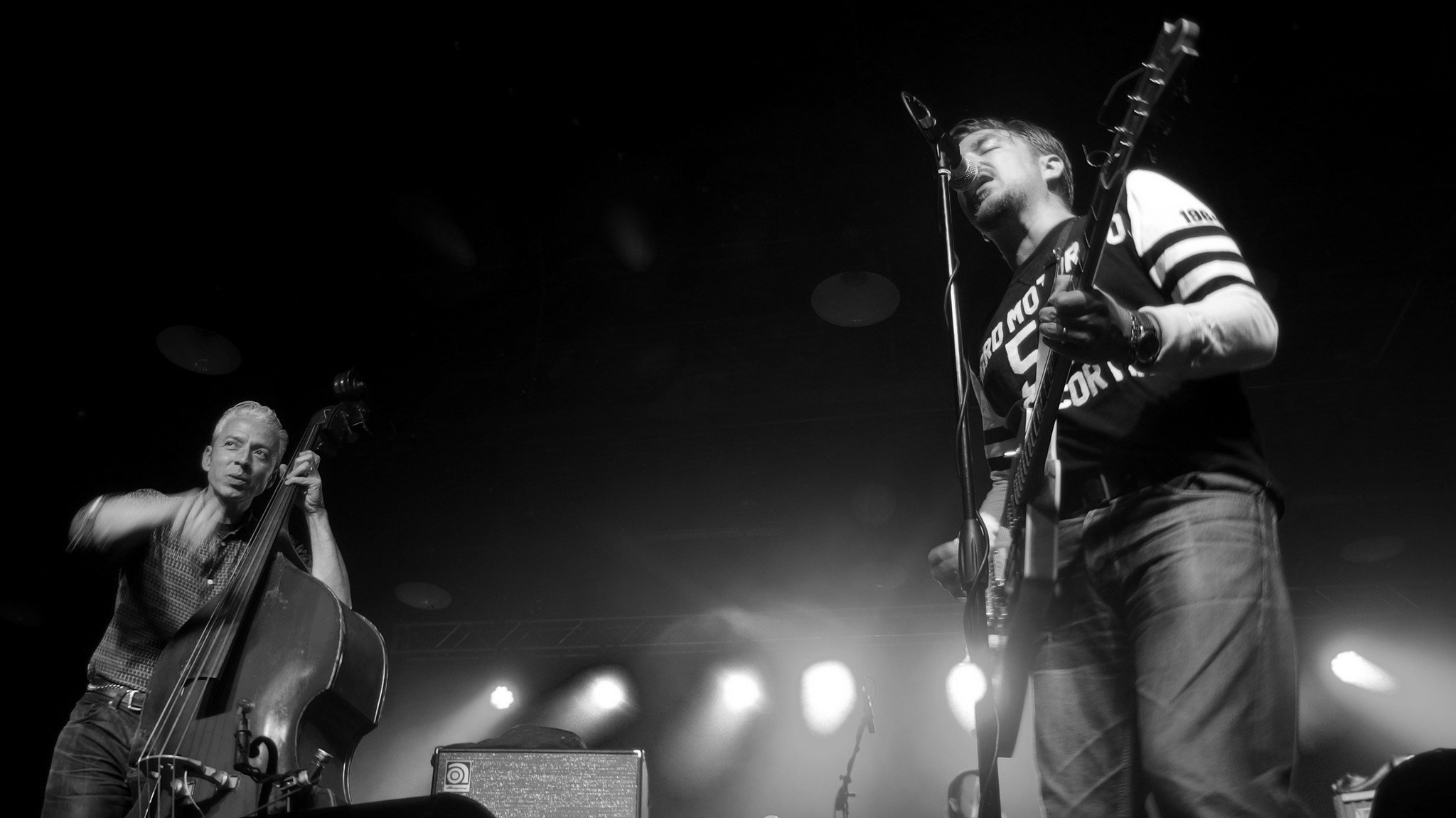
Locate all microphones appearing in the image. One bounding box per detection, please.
[901,88,980,193]
[859,682,876,733]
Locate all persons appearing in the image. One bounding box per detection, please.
[44,400,353,818]
[927,116,1303,818]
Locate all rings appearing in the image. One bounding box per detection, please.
[1062,327,1066,333]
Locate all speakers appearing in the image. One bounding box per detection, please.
[427,742,649,818]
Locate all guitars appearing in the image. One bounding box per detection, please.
[957,18,1207,761]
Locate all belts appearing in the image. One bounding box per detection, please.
[86,676,147,710]
[1060,465,1190,521]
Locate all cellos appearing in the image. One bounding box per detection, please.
[118,366,392,817]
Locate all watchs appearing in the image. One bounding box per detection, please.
[1123,311,1160,367]
